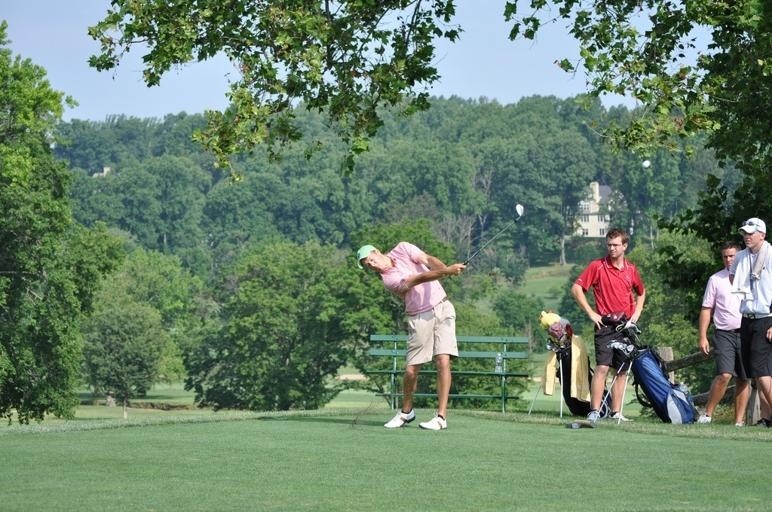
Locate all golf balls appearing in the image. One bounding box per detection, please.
[642,160,651,169]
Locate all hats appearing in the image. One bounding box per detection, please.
[738,217,766,235]
[357,244,376,269]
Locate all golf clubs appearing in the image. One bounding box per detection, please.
[546,334,569,357]
[606,338,638,358]
[460,204,524,269]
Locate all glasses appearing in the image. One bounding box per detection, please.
[742,221,759,226]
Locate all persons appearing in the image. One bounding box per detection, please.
[698,241,752,426]
[728,218,772,426]
[571,229,645,428]
[358,241,470,429]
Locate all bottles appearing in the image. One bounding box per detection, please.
[494,351,503,374]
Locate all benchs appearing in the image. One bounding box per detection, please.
[364,331,531,416]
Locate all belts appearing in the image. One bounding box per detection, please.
[439,297,448,303]
[714,328,741,334]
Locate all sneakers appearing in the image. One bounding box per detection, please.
[384,409,415,428]
[418,417,448,430]
[608,412,633,422]
[697,414,712,423]
[586,410,601,421]
